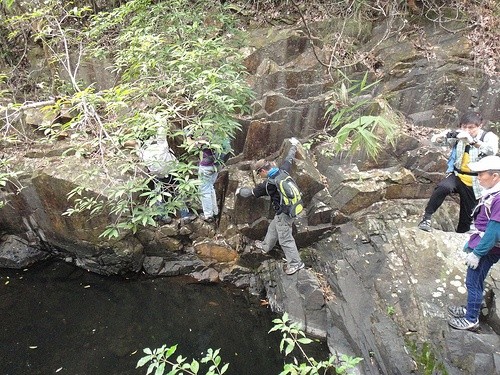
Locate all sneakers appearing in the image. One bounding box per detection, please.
[447,305,467,318]
[417,214,432,232]
[255,239,267,253]
[285,262,305,274]
[449,316,479,330]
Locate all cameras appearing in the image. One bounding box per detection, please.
[447,132,459,138]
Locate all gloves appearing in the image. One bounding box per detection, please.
[464,253,481,270]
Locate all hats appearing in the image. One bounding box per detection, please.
[469,156,500,171]
[255,159,266,174]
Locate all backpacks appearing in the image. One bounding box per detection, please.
[265,169,304,218]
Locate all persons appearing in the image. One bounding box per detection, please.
[418,112,500,233]
[195,137,220,223]
[449,154,500,330]
[234,161,305,275]
[125,117,193,223]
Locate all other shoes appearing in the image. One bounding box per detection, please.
[205,215,218,223]
[161,215,171,223]
[182,214,191,220]
